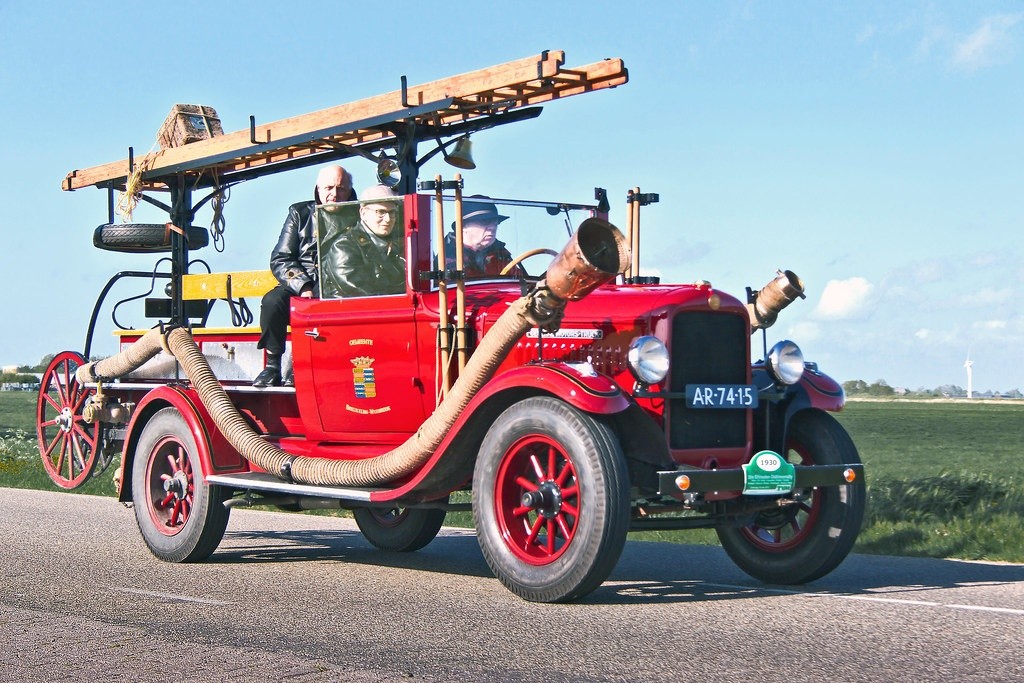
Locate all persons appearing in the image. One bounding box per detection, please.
[324,186,408,298]
[252,164,362,389]
[435,195,521,284]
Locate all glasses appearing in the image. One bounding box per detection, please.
[366,208,399,219]
[468,218,499,226]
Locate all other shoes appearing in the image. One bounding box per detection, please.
[284,368,294,387]
[253,368,282,386]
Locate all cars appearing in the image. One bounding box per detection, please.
[117,200,868,608]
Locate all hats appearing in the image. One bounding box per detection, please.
[451,195,510,232]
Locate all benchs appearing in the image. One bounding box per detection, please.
[110,272,292,383]
